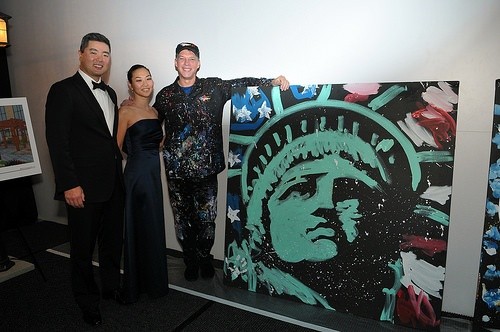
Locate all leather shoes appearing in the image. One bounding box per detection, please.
[82,305,105,328]
[101,288,128,306]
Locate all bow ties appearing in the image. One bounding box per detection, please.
[92,80,106,92]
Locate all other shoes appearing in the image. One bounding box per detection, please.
[184,265,199,282]
[200,262,215,279]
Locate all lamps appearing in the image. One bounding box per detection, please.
[0,11,14,48]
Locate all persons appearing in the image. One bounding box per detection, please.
[117,64,168,296]
[45,33,134,326]
[120,43,289,281]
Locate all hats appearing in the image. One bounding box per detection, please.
[176,43,199,58]
